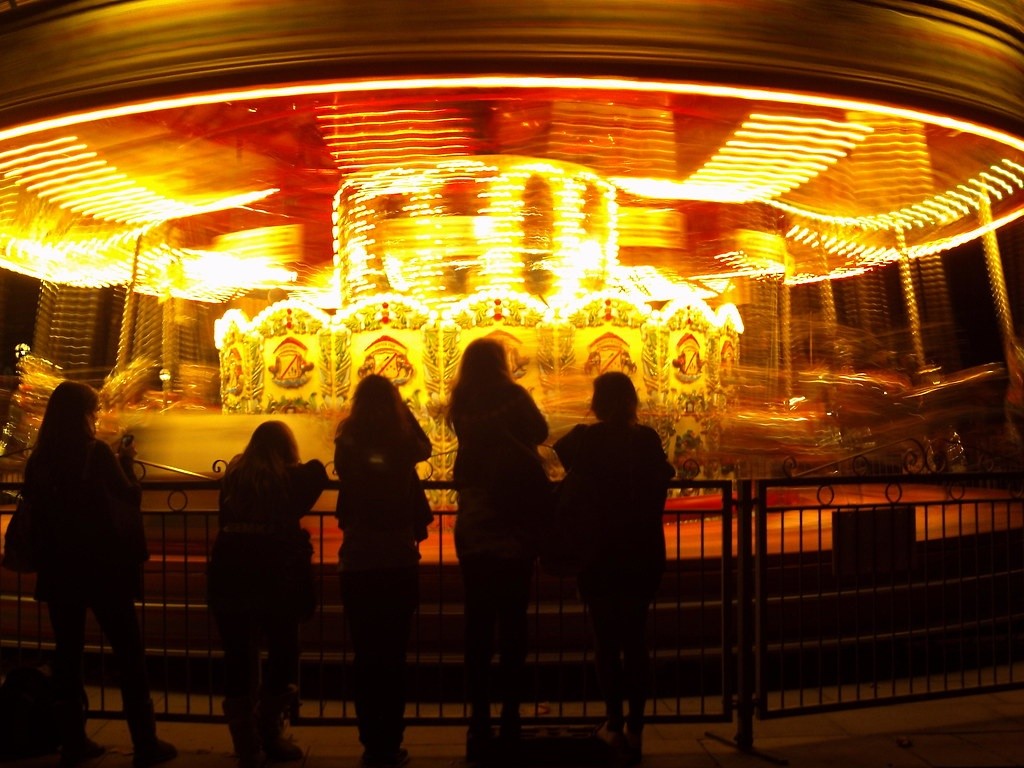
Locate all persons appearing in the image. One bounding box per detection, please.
[334,374,435,768]
[207,422,328,768]
[444,339,553,730]
[553,372,677,746]
[21,382,179,768]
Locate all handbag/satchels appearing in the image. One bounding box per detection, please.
[0,664,89,759]
[2,502,50,573]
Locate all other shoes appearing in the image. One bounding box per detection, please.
[498,708,521,732]
[596,721,624,752]
[623,723,642,751]
[470,707,491,732]
[362,748,409,768]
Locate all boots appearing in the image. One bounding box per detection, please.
[222,695,268,768]
[50,695,105,760]
[124,700,177,765]
[260,694,304,760]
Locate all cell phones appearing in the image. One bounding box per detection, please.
[117,434,134,455]
